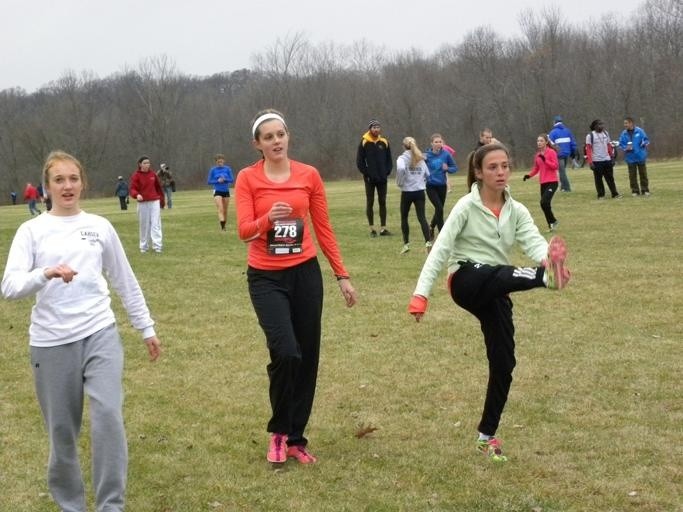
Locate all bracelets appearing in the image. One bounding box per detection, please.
[337,277,349,282]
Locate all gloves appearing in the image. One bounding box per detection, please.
[538,154,544,161]
[523,175,528,180]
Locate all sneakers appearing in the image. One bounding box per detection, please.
[546,235,566,290]
[475,436,507,463]
[597,189,650,201]
[286,445,315,464]
[267,433,287,463]
[549,220,559,232]
[371,228,434,256]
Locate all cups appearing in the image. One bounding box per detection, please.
[626,141,633,150]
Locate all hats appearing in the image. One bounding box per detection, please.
[551,115,560,121]
[368,118,380,128]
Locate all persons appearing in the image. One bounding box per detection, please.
[523,134,560,234]
[207,154,236,231]
[570,146,580,170]
[156,163,176,210]
[233,108,357,465]
[549,115,577,192]
[406,142,571,461]
[581,143,589,169]
[619,117,651,197]
[113,175,131,211]
[11,182,53,216]
[356,119,501,255]
[0,150,161,512]
[127,155,166,254]
[586,119,620,199]
